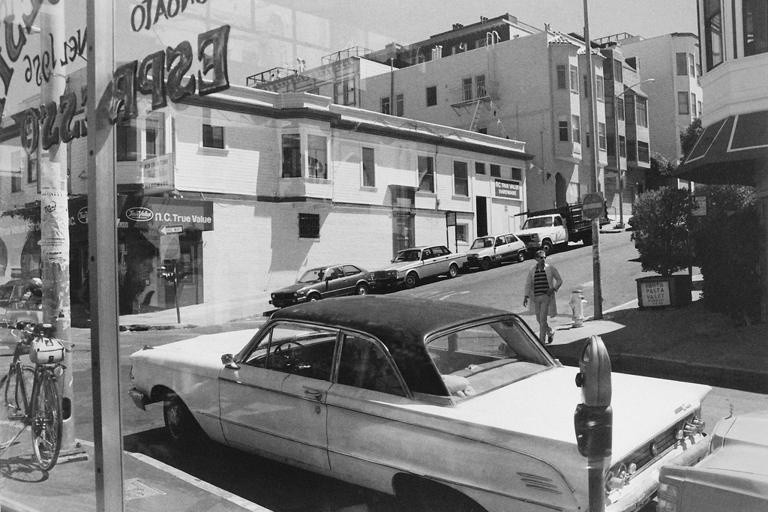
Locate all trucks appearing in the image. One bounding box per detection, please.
[517,200,609,255]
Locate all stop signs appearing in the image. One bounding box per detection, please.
[582,193,604,218]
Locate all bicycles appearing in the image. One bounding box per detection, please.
[1,321,65,474]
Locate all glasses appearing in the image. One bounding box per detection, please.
[540,257,546,259]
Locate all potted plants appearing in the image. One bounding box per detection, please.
[626,185,700,310]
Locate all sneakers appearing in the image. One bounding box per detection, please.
[548,330,555,344]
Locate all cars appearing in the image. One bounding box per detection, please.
[131,298,712,512]
[469,232,529,270]
[368,244,468,288]
[271,265,374,306]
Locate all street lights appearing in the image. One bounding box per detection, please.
[611,78,657,229]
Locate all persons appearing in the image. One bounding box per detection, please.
[522,249,562,345]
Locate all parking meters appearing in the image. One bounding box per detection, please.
[573,336,613,512]
